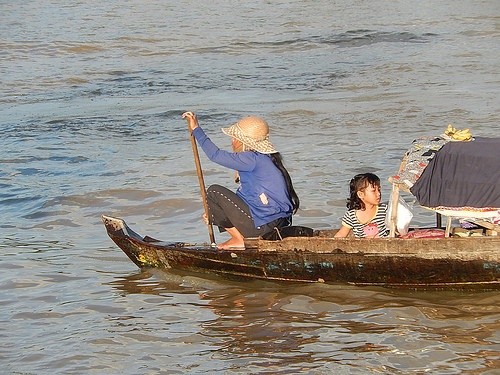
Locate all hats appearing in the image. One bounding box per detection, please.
[221,117,278,154]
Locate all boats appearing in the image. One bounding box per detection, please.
[100,134,500,291]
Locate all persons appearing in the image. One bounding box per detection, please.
[182,111,299,250]
[333,172,401,239]
